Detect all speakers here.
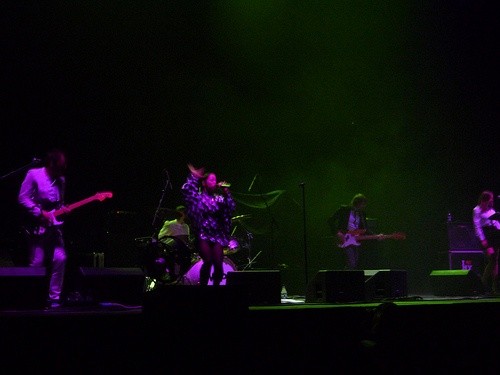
[78,266,145,307]
[226,270,282,307]
[305,269,408,305]
[429,221,488,297]
[0,266,49,311]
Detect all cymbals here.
[231,215,252,222]
[223,247,241,256]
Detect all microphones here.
[248,175,258,192]
[218,183,232,188]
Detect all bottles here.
[447,212,452,222]
[460,258,472,270]
[87,248,104,268]
[280,283,287,299]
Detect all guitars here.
[25,192,112,235]
[336,230,407,249]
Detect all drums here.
[183,258,239,285]
[158,235,192,258]
[152,258,180,281]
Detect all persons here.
[181,164,236,285]
[18,151,73,308]
[327,193,368,270]
[472,191,500,293]
[158,205,193,243]
